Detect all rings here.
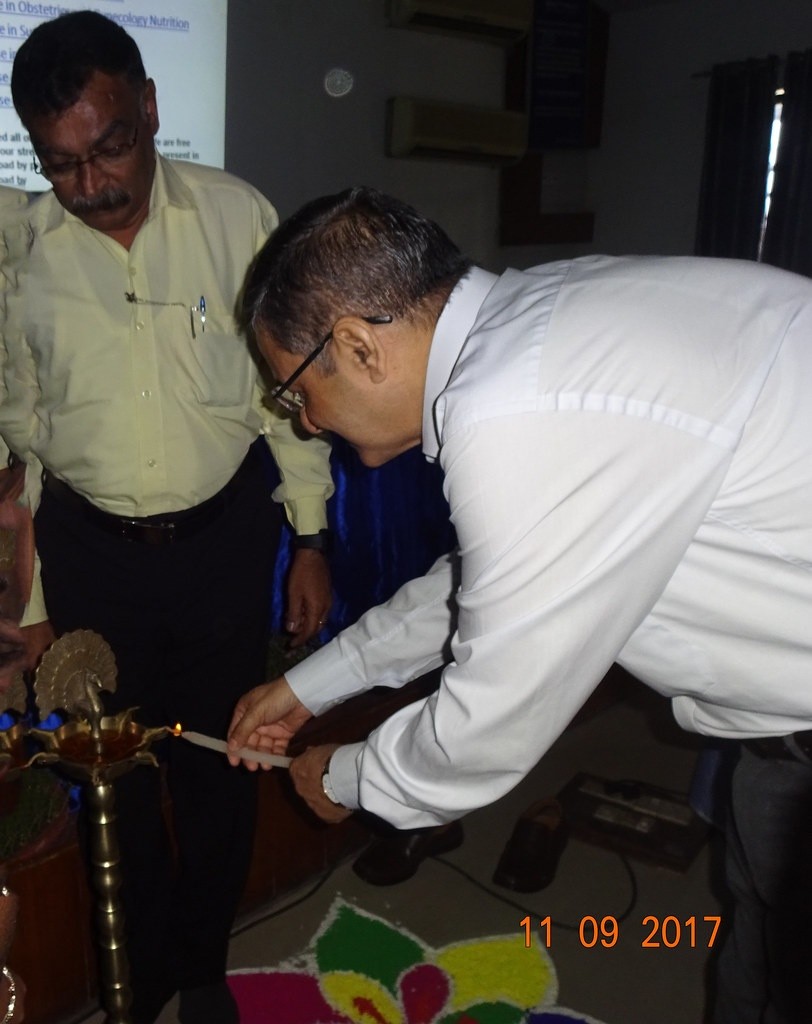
[318,621,326,624]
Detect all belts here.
[44,444,261,546]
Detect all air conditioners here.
[384,95,530,167]
[383,0,534,48]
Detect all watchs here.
[321,755,351,811]
[294,529,335,561]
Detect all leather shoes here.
[494,797,577,892]
[351,821,462,885]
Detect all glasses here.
[271,314,392,413]
[33,88,144,183]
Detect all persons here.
[227,188,812,1024]
[0,10,335,1023]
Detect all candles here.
[178,730,293,768]
